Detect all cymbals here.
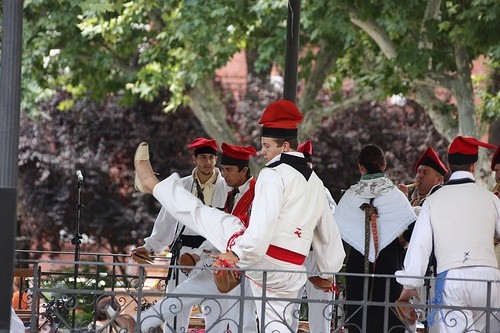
[214,267,241,293]
[395,301,418,326]
[131,248,153,265]
[180,253,195,273]
[308,276,332,291]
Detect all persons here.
[491,142,500,271]
[394,135,500,333]
[88,297,121,333]
[397,146,448,333]
[337,144,416,333]
[134,100,346,333]
[131,138,229,333]
[106,141,257,333]
[291,139,338,333]
[12,220,31,310]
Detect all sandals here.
[134,141,150,194]
[104,304,130,333]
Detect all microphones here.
[76,170,83,184]
[195,178,206,205]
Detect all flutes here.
[398,182,420,189]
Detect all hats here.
[221,142,257,168]
[415,146,448,177]
[258,100,303,139]
[491,144,500,171]
[447,136,497,166]
[187,138,218,158]
